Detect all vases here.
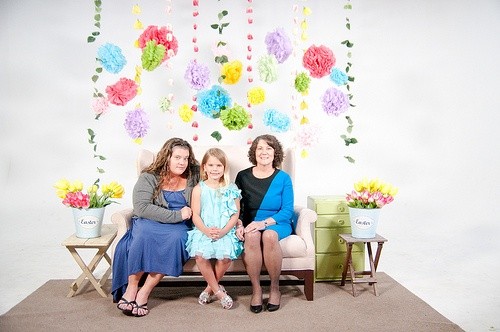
[72,207,105,239]
[349,207,380,239]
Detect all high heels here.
[249,288,263,314]
[267,290,282,312]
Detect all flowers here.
[54,178,124,210]
[346,181,398,209]
[87,0,359,173]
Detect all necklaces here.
[171,179,180,190]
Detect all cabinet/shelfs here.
[306,196,365,282]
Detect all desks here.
[62,227,118,298]
[338,233,388,298]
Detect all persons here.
[235,135,297,314]
[111,137,202,317]
[185,148,243,310]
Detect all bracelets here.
[264,220,267,227]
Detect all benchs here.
[111,206,317,301]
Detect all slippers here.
[116,297,149,318]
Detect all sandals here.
[214,284,233,310]
[197,288,212,306]
